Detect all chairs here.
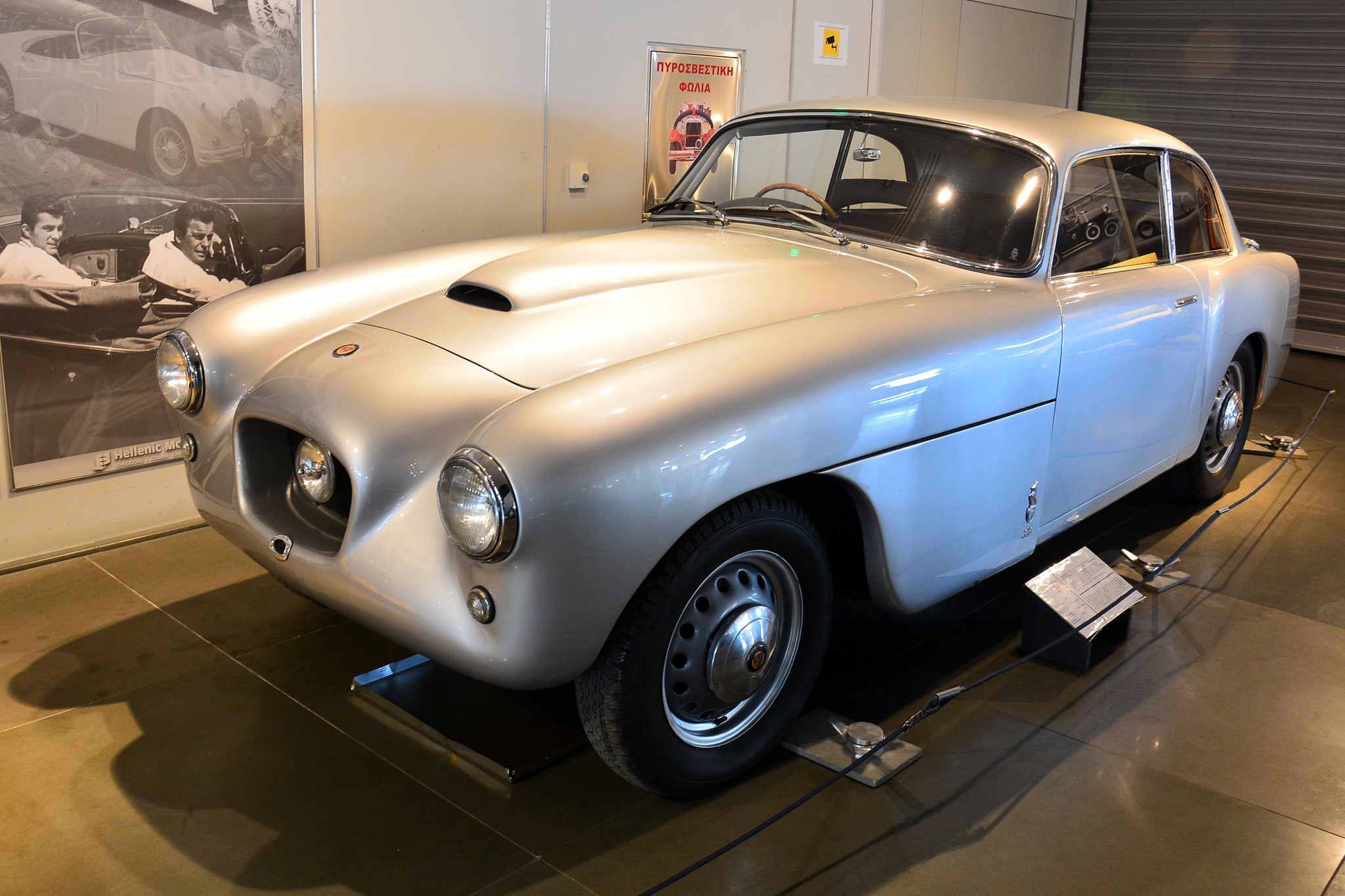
[913,191,1029,265]
[829,177,915,234]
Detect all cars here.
[0,1,304,187]
[151,90,1303,804]
[0,185,306,454]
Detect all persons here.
[0,194,117,288]
[140,200,251,305]
